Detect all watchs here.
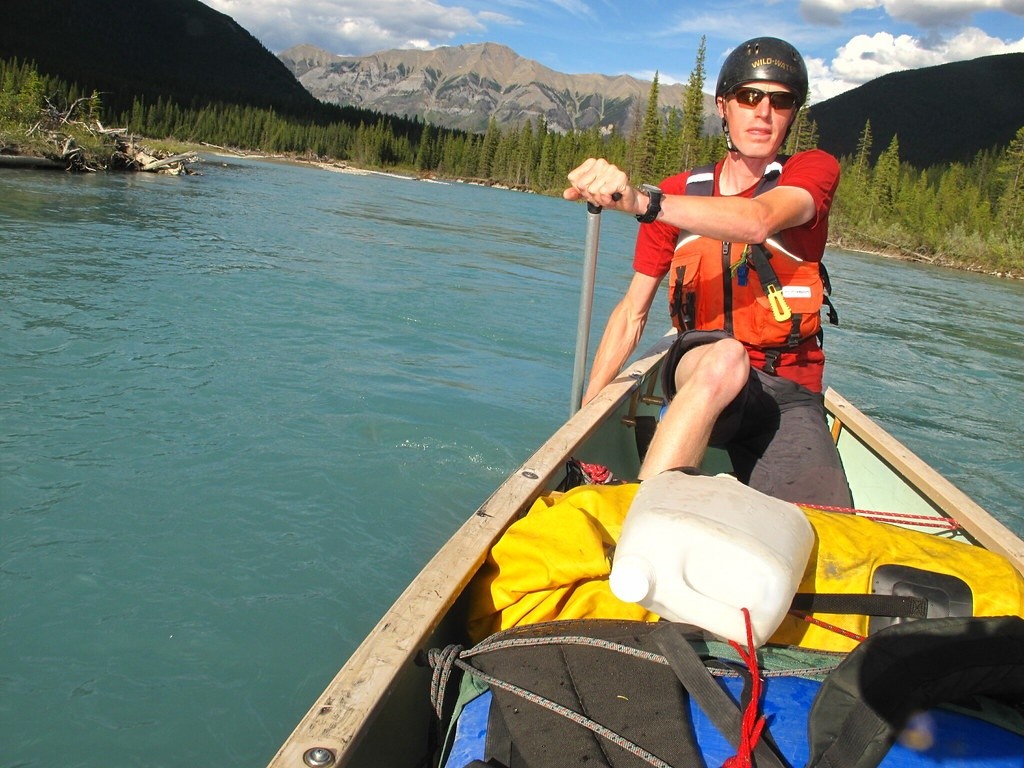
[635,184,663,223]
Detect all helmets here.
[716,37,809,108]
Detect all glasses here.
[731,86,797,108]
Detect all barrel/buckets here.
[609,471,815,650]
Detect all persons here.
[563,38,855,515]
